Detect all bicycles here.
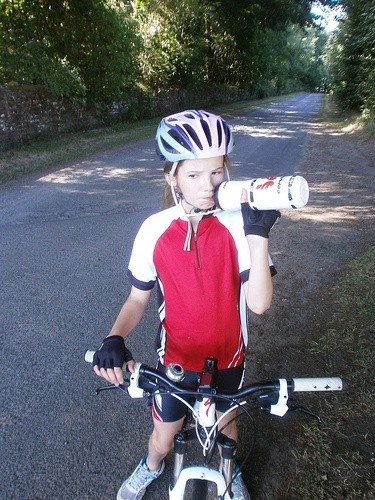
[85,349,345,500]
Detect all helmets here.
[155,110,235,161]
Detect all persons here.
[91,108,283,500]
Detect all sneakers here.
[116,455,164,500]
[219,459,251,499]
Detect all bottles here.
[214,174,312,212]
